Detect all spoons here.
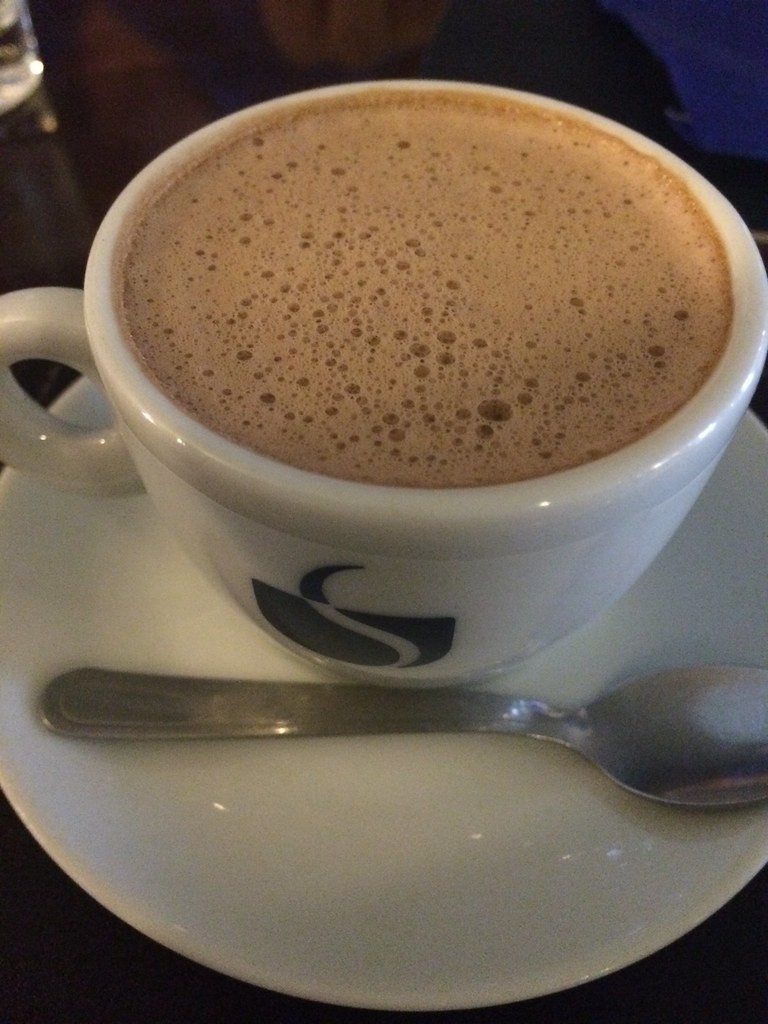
[37,664,768,809]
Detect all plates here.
[0,373,768,1011]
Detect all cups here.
[1,3,42,109]
[1,79,768,692]
